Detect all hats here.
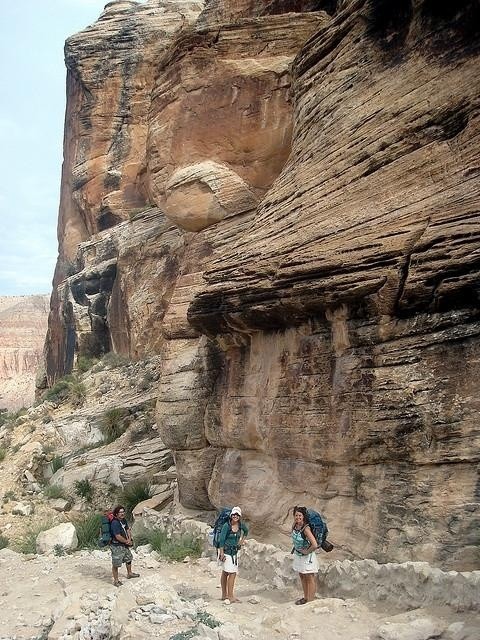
[230,506,242,518]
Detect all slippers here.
[222,597,241,603]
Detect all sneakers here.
[295,598,307,605]
[114,580,123,586]
[127,573,139,579]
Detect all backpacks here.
[213,508,241,551]
[292,508,328,549]
[97,514,120,547]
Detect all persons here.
[108,505,139,587]
[217,506,249,604]
[290,504,320,605]
